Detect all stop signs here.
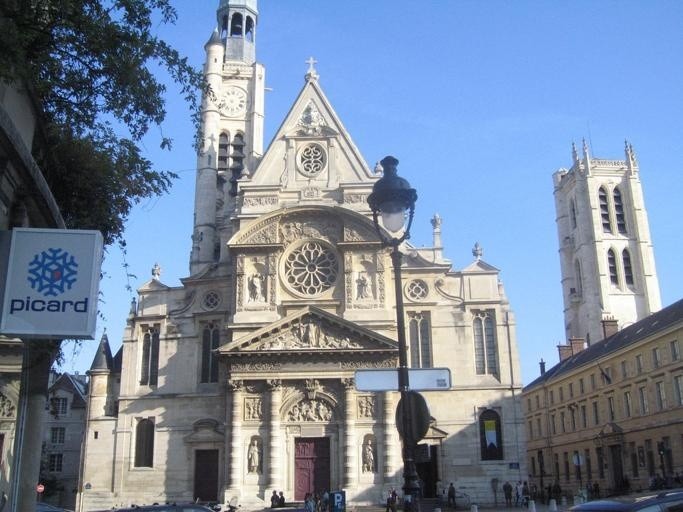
[37,485,44,493]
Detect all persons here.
[247,401,254,420]
[290,398,333,421]
[435,478,443,496]
[1,489,8,509]
[501,478,599,509]
[248,439,262,474]
[363,438,376,472]
[447,482,456,508]
[386,485,395,512]
[258,401,264,418]
[369,398,378,416]
[271,490,279,507]
[356,275,371,300]
[304,485,330,512]
[278,491,285,506]
[247,271,265,302]
[390,490,400,512]
[645,470,683,491]
[360,400,367,417]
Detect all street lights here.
[367,156,421,511]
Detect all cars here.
[569,490,683,511]
[110,504,220,512]
